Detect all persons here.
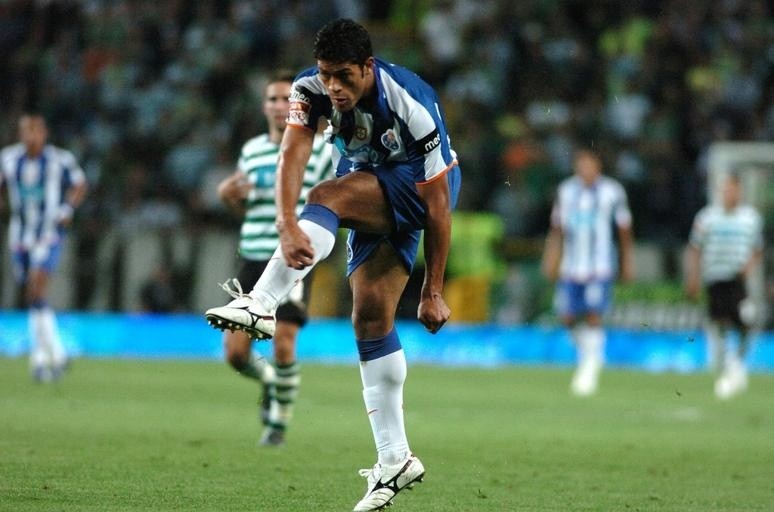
[201,19,461,511]
[679,167,765,397]
[218,73,337,449]
[538,140,636,398]
[0,0,773,333]
[1,112,87,383]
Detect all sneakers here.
[204,292,278,343]
[259,425,285,446]
[353,450,426,512]
[258,364,279,426]
